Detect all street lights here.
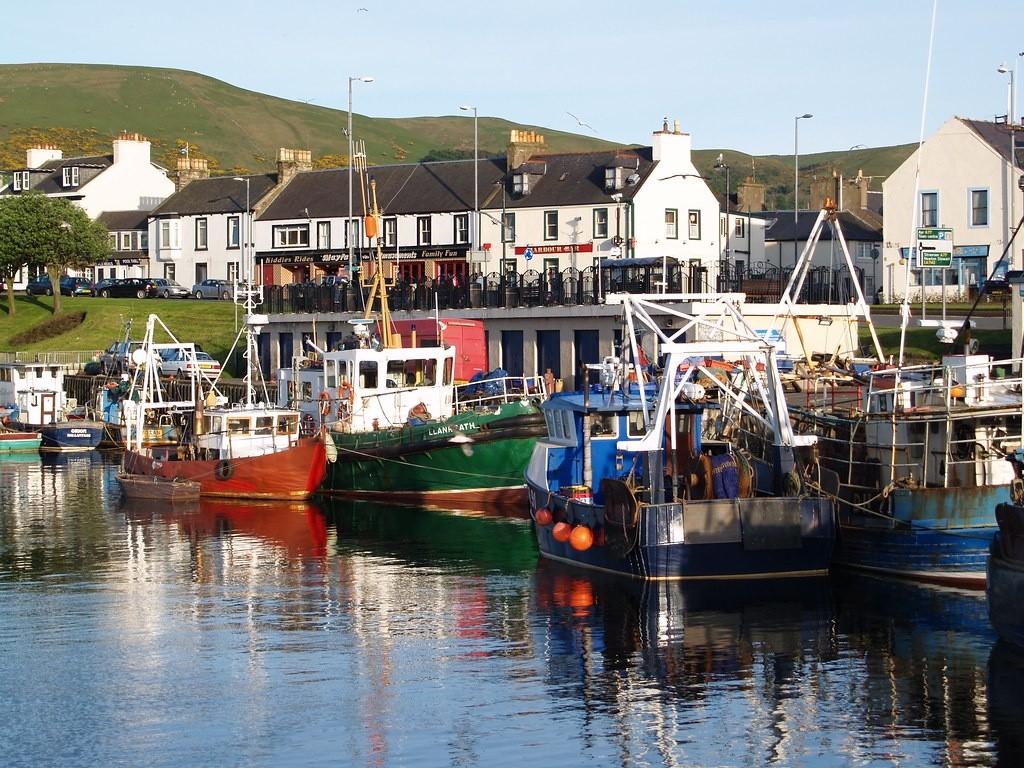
[996,62,1018,275]
[348,75,373,281]
[793,114,814,280]
[715,163,733,294]
[233,176,259,285]
[460,105,485,276]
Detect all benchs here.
[740,280,786,298]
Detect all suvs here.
[26,273,70,296]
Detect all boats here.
[1,415,42,452]
[121,310,332,506]
[525,279,845,607]
[772,203,1023,633]
[268,244,552,524]
[159,494,329,565]
[1,451,44,465]
[117,497,202,515]
[42,451,104,468]
[0,365,106,452]
[315,501,538,573]
[115,474,203,499]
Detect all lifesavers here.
[318,392,330,416]
[302,414,315,434]
[339,382,354,405]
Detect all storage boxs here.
[560,486,592,504]
[630,350,792,404]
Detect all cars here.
[152,279,191,299]
[92,278,123,298]
[52,277,94,297]
[193,278,245,301]
[103,279,158,298]
